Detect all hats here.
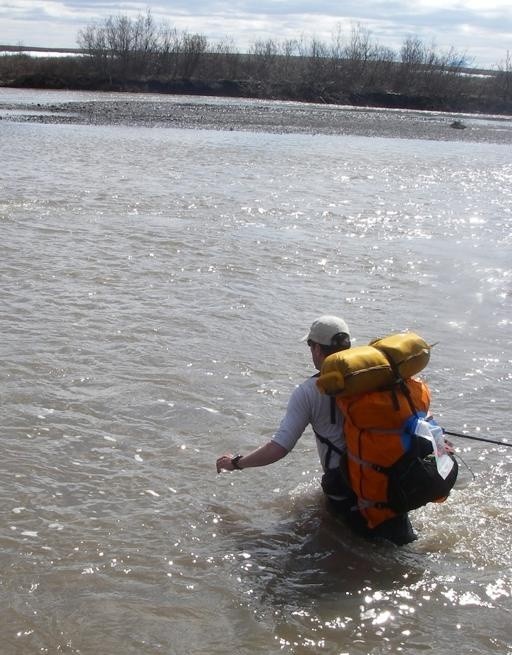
[298,312,351,349]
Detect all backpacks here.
[310,370,460,533]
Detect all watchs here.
[230,454,244,469]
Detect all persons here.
[216,314,455,545]
